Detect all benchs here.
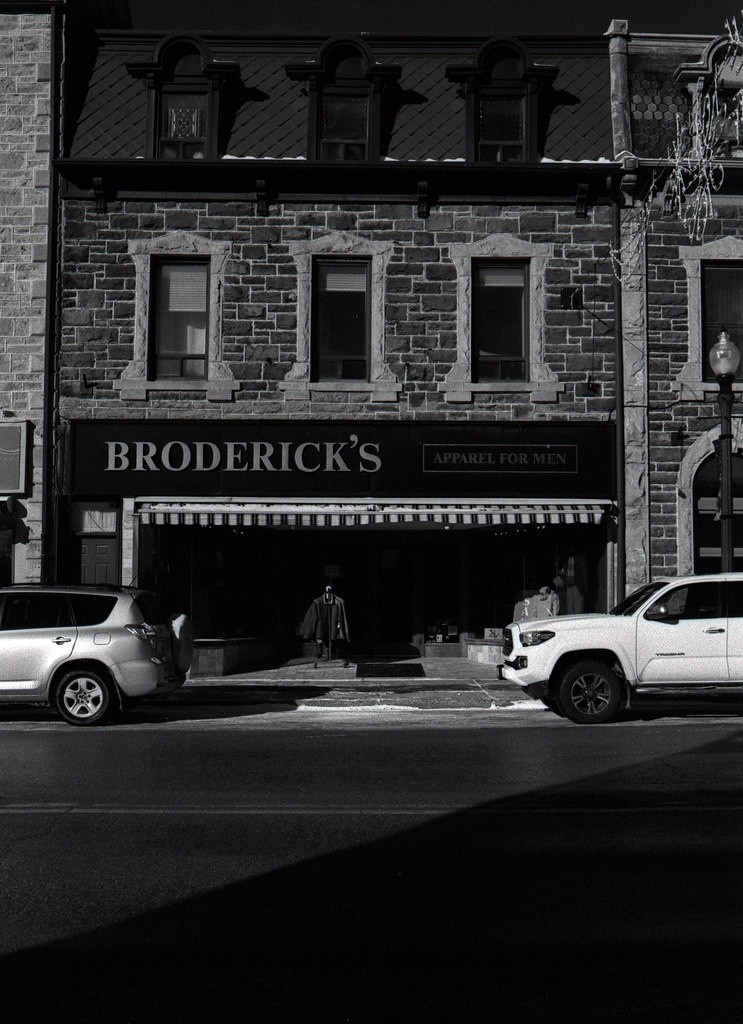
[190,637,256,678]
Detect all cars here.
[496,573,743,724]
[0,582,194,726]
